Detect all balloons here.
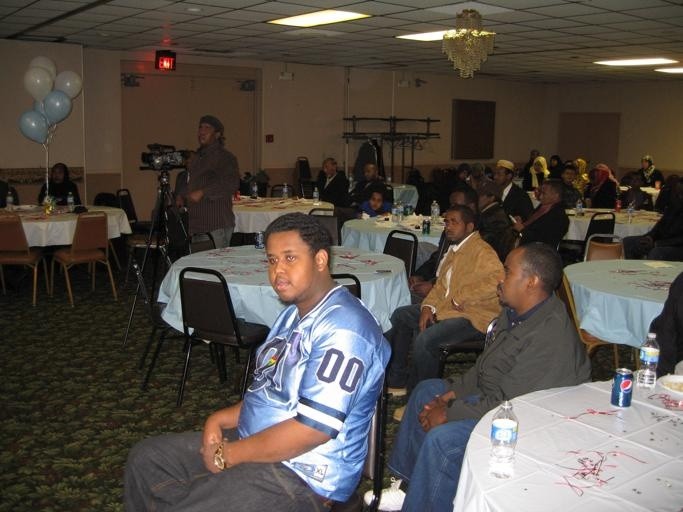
[16,54,83,145]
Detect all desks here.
[562,259,682,366]
[158,244,414,354]
[182,195,334,237]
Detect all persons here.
[382,207,506,421]
[448,149,618,247]
[386,184,501,388]
[360,240,592,512]
[619,173,654,210]
[172,149,197,238]
[622,178,682,261]
[642,271,683,384]
[635,155,664,186]
[0,180,9,208]
[36,161,84,205]
[316,157,393,219]
[172,115,239,252]
[120,210,393,511]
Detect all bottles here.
[6,191,13,212]
[281,181,287,200]
[627,203,634,223]
[636,332,660,394]
[575,198,582,216]
[391,198,440,225]
[313,186,319,204]
[66,192,75,212]
[250,181,260,198]
[489,396,518,479]
[615,193,622,212]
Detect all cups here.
[48,195,57,210]
[654,181,661,191]
[45,199,52,215]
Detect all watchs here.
[212,439,229,472]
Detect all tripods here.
[121,170,193,348]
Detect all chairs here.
[130,258,224,390]
[49,209,118,308]
[1,213,50,307]
[270,182,517,278]
[326,352,389,511]
[435,338,484,381]
[383,229,419,280]
[559,272,641,369]
[185,231,217,255]
[2,189,141,227]
[308,208,343,247]
[517,155,681,261]
[330,272,362,300]
[173,266,272,407]
[122,210,174,288]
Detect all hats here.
[498,160,514,170]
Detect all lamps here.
[441,7,495,81]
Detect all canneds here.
[404,204,413,216]
[422,219,430,234]
[255,231,265,249]
[611,368,633,407]
[234,190,241,201]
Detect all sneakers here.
[364,479,408,511]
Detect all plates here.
[657,373,682,396]
